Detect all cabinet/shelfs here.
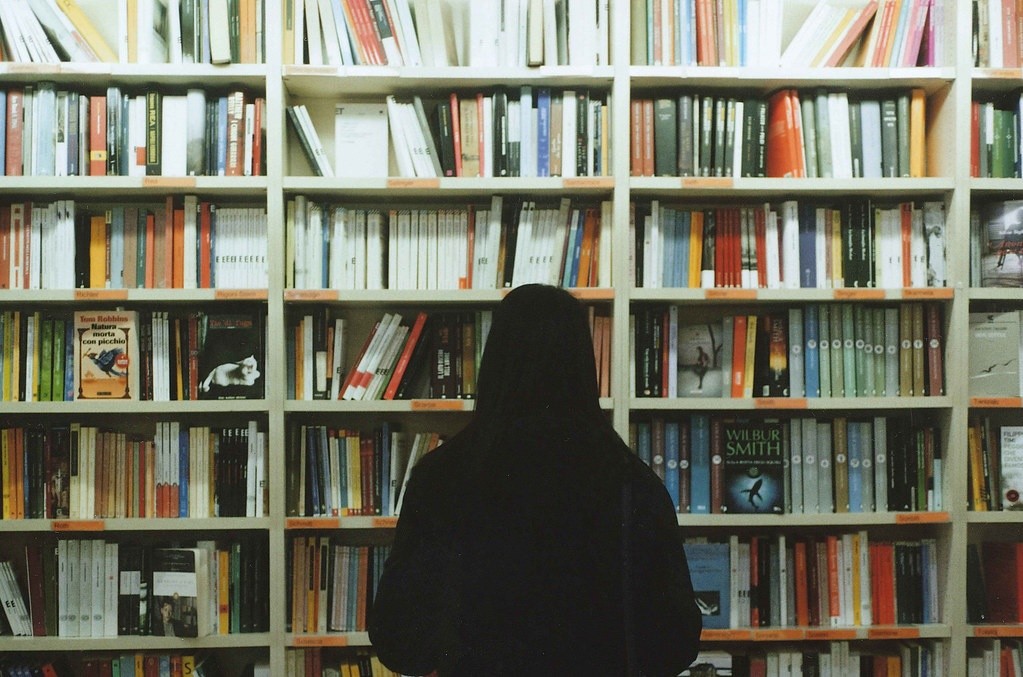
[1,0,1023,676]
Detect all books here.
[0,0,1023,677]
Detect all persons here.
[370,284,703,677]
